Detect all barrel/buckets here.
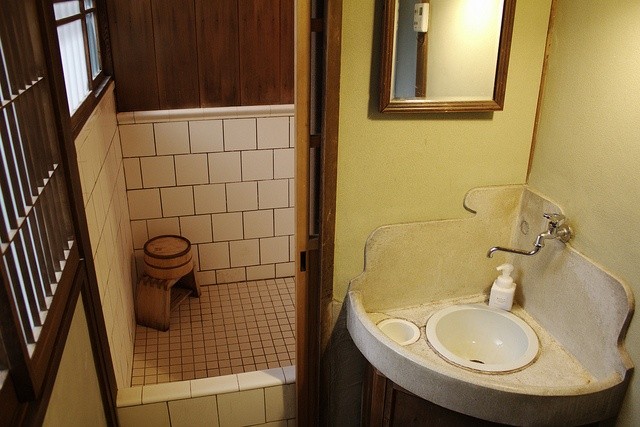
[143,234,193,279]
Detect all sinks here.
[426,304,539,373]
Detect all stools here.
[135,263,202,332]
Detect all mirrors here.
[377,0,516,115]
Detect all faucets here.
[487,213,573,258]
